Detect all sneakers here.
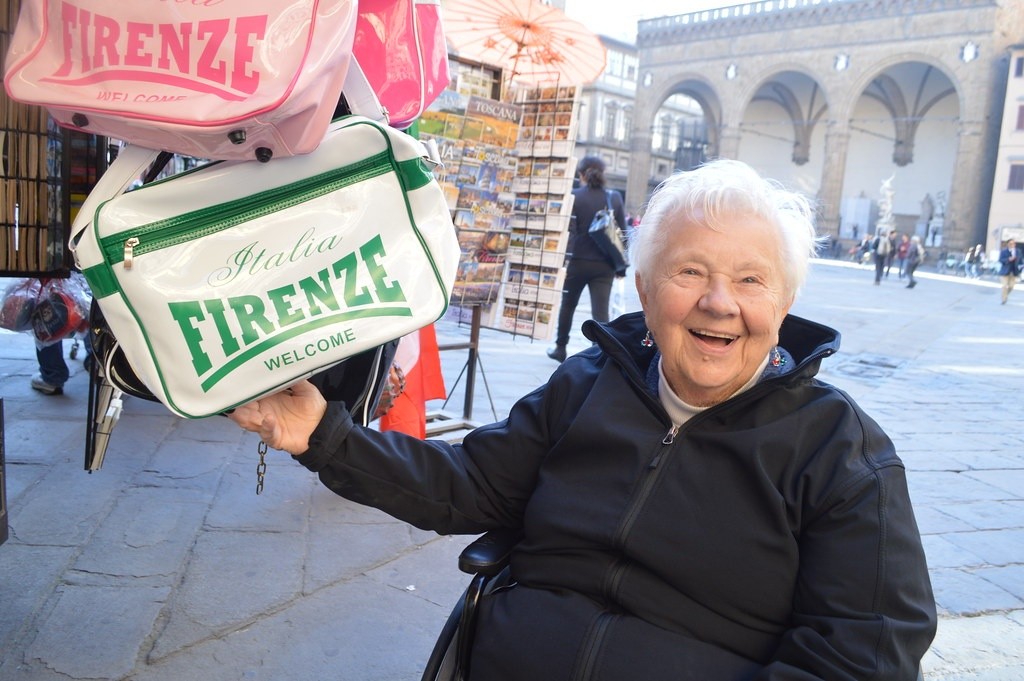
[31,371,63,394]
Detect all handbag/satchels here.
[588,187,630,274]
[65,53,460,420]
[0,271,92,343]
[3,1,359,162]
[93,320,406,430]
[353,0,451,129]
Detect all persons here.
[871,231,921,288]
[965,244,982,280]
[855,235,875,265]
[998,239,1022,304]
[548,156,632,362]
[626,212,641,226]
[233,158,939,681]
[31,278,93,395]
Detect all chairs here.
[421,527,526,681]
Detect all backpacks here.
[877,236,891,256]
[916,244,925,265]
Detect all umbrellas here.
[441,0,607,101]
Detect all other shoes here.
[875,273,882,282]
[1007,285,1013,294]
[906,281,916,288]
[1001,297,1008,304]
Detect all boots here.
[546,342,566,363]
